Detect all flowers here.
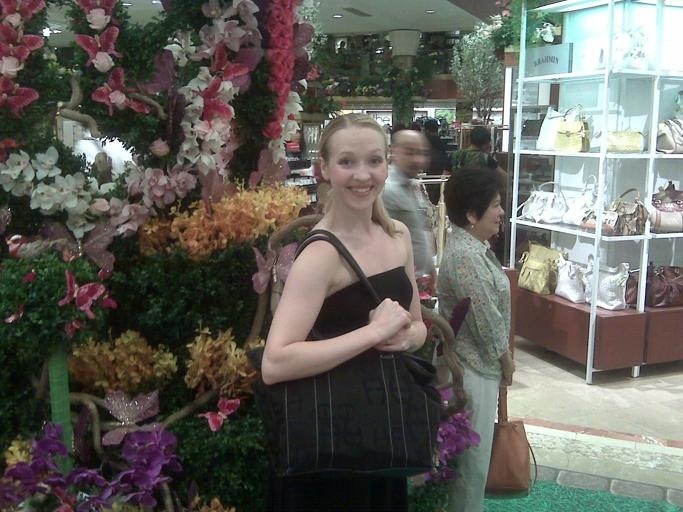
[0,0,320,470]
[489,0,512,48]
[68,185,316,511]
[431,410,481,484]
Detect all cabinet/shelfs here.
[510,0,683,384]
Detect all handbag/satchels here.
[535,103,583,151]
[625,264,682,309]
[252,231,441,484]
[560,174,607,226]
[605,129,644,153]
[515,178,569,224]
[554,248,590,304]
[650,180,683,234]
[517,251,549,295]
[656,118,683,154]
[584,258,630,311]
[528,240,560,290]
[579,206,620,235]
[603,187,648,237]
[482,380,536,496]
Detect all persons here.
[381,129,436,303]
[436,166,514,512]
[443,127,509,177]
[262,114,439,512]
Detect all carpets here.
[482,478,683,511]
